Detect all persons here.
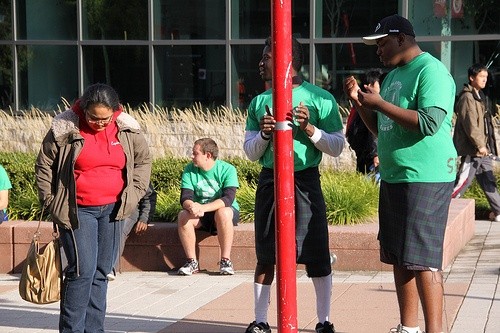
[0,163,13,224]
[35,84,156,333]
[346,17,456,333]
[243,36,346,333]
[348,64,500,224]
[177,138,240,275]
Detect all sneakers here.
[177,258,199,276]
[217,258,234,275]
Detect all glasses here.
[86,110,114,123]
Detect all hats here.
[362,14,416,46]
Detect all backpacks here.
[345,103,372,155]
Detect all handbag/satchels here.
[19,194,63,304]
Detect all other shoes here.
[315,321,335,333]
[245,321,271,333]
[388,324,425,333]
